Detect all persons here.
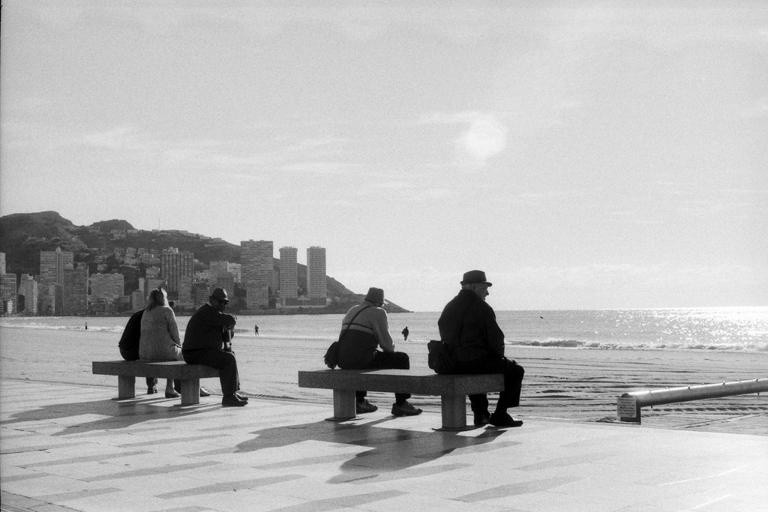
[438,270,525,428]
[182,287,248,407]
[402,326,409,341]
[335,287,423,417]
[254,324,259,336]
[138,288,210,398]
[118,300,181,395]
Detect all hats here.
[363,287,389,305]
[459,269,492,288]
[208,286,230,303]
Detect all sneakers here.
[199,387,211,397]
[390,402,422,415]
[147,384,158,395]
[356,400,378,414]
[222,391,249,406]
[165,387,181,399]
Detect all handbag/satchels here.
[323,341,340,369]
[427,338,459,375]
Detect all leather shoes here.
[473,411,490,427]
[489,412,523,427]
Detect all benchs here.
[297,367,505,432]
[91,359,220,406]
